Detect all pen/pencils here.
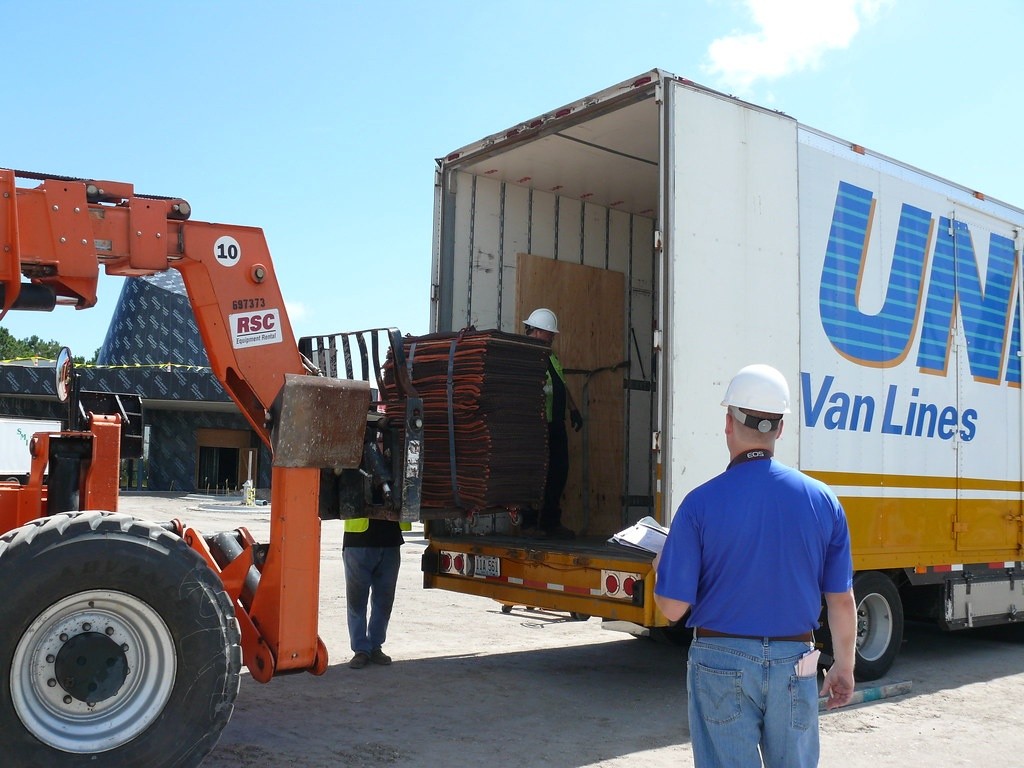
[822,667,835,699]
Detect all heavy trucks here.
[419,67,1023,684]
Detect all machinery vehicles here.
[1,163,539,768]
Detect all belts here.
[694,625,815,641]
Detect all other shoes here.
[369,650,392,665]
[349,652,370,669]
[542,521,577,539]
[515,522,552,540]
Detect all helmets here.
[522,308,560,334]
[720,363,792,417]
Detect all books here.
[604,515,671,558]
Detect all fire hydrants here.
[241,479,253,506]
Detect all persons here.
[649,363,859,768]
[343,517,411,669]
[521,307,585,541]
